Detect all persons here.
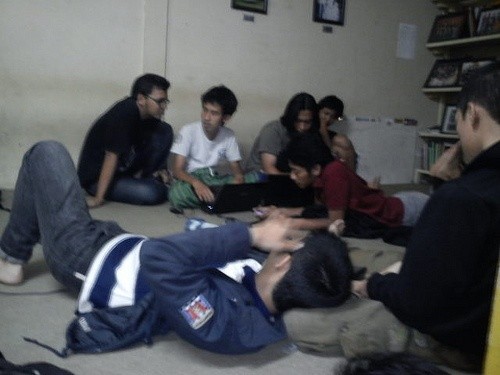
[77,73,174,207]
[244,63,500,375]
[169,84,243,211]
[0,140,354,357]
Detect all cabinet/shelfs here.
[416,0,500,190]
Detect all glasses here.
[144,94,170,106]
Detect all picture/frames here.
[441,103,458,134]
[474,7,500,35]
[424,60,462,87]
[313,0,346,26]
[428,11,467,41]
[230,0,268,14]
[458,58,497,88]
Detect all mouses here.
[170,207,184,214]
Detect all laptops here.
[200,182,270,214]
